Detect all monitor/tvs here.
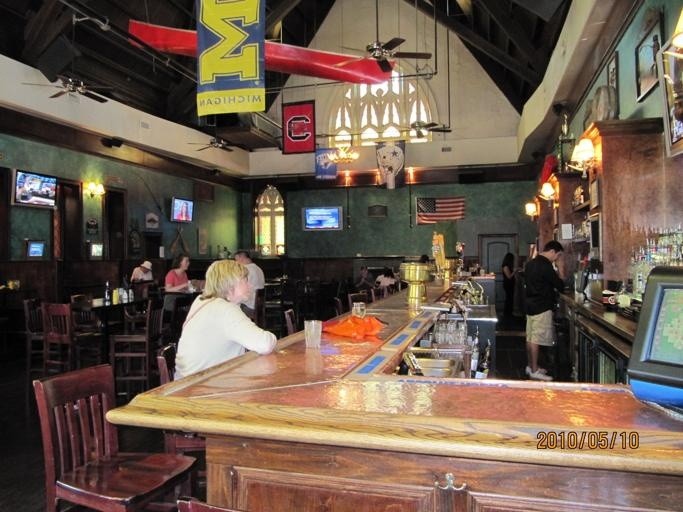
[301,206,343,231]
[626,266,682,408]
[12,168,59,209]
[171,196,195,223]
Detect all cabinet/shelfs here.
[537,117,683,383]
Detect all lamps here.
[325,143,361,164]
[84,181,106,199]
[538,182,555,208]
[525,202,537,222]
[670,8,683,50]
[569,138,596,179]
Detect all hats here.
[140,261,152,271]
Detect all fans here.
[22,16,116,106]
[186,126,247,152]
[330,0,433,74]
[410,121,452,139]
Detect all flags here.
[374,139,407,190]
[282,100,318,155]
[313,149,338,180]
[414,196,466,227]
[195,0,267,117]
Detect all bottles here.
[627,224,683,302]
[104,280,111,308]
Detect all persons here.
[163,253,199,312]
[167,257,281,381]
[501,251,523,320]
[374,266,397,292]
[352,263,376,293]
[130,260,154,284]
[233,250,267,315]
[522,240,569,381]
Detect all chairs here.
[32,364,198,512]
[70,294,97,330]
[23,298,62,369]
[156,343,207,501]
[109,298,165,372]
[170,294,196,333]
[348,293,369,312]
[176,496,240,512]
[334,297,343,316]
[284,308,297,336]
[371,288,385,302]
[262,283,294,329]
[72,305,108,369]
[40,302,72,375]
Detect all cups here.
[304,320,322,347]
[351,302,365,318]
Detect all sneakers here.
[529,371,554,382]
[525,367,548,374]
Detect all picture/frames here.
[655,37,683,160]
[633,14,665,104]
[606,50,620,115]
